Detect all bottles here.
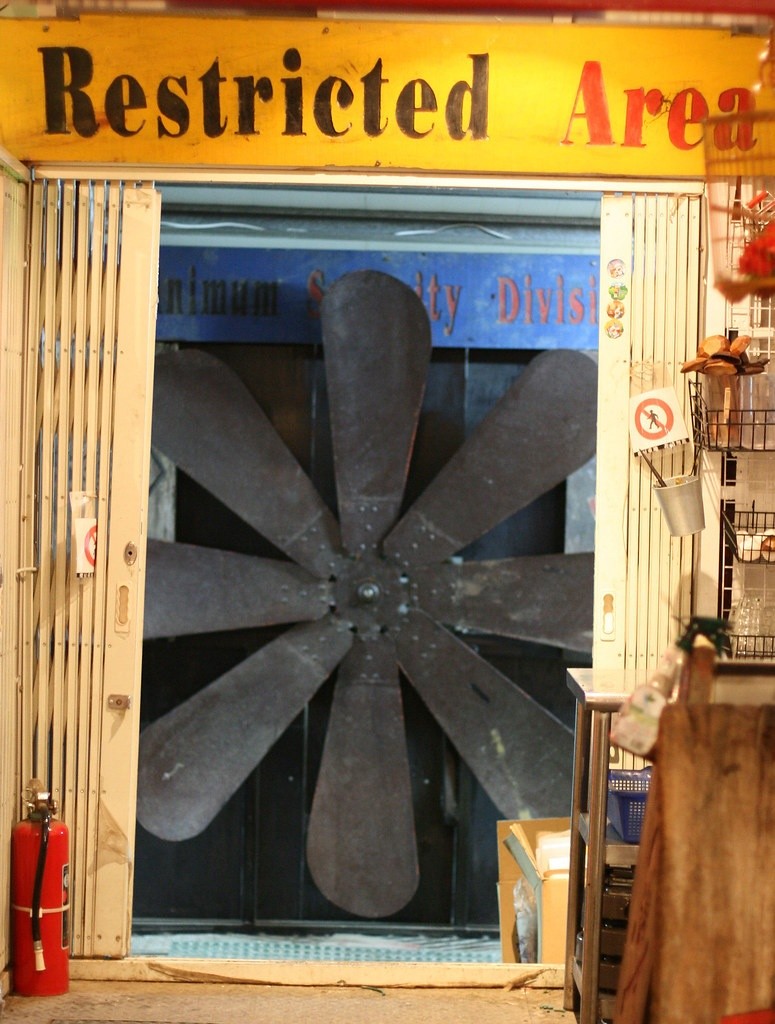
[608,674,673,758]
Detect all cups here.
[739,373,766,443]
[705,374,737,446]
[731,591,768,649]
[653,476,704,536]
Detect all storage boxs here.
[497,817,572,965]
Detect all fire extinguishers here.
[11,778,72,998]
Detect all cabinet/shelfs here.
[563,668,654,1024]
[687,378,775,677]
